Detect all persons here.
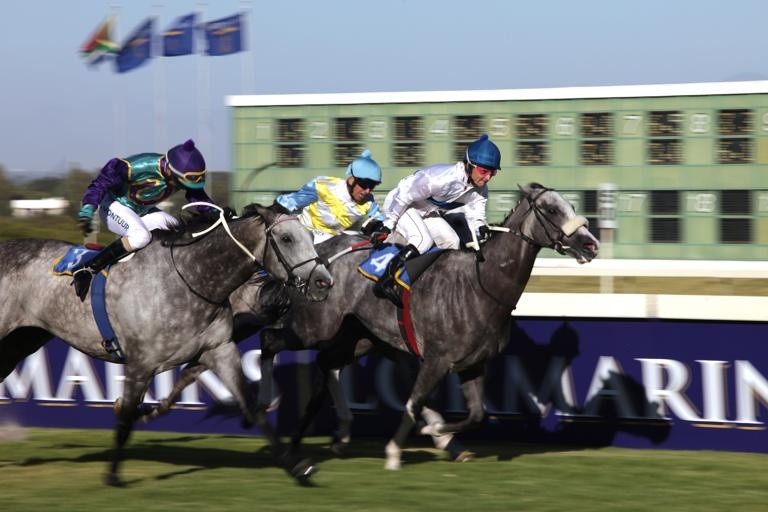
[272,151,387,245]
[372,135,501,309]
[71,141,235,296]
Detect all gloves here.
[369,225,391,250]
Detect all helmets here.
[345,149,382,185]
[461,133,502,171]
[164,139,205,191]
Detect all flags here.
[81,15,118,69]
[203,12,243,56]
[162,14,194,55]
[113,16,151,73]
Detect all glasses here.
[354,177,378,190]
[171,168,208,184]
[469,164,497,177]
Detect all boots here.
[71,235,130,298]
[372,245,419,308]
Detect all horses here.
[114,270,477,463]
[242,182,600,473]
[0,200,334,487]
